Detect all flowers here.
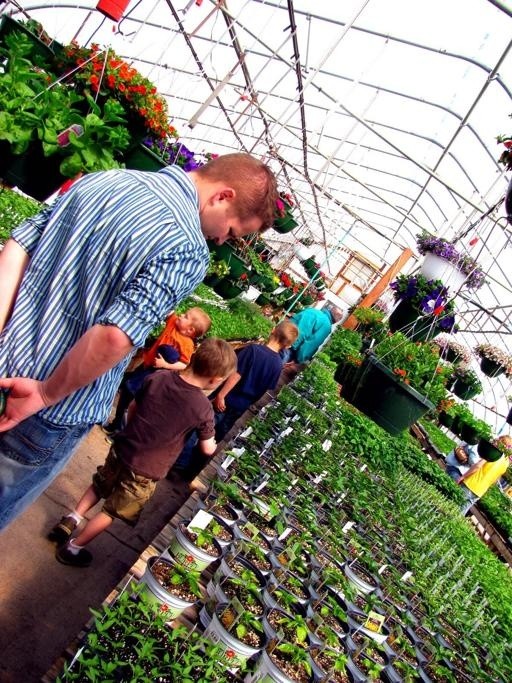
[0,14,512,683]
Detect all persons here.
[45,334,239,570]
[1,151,284,536]
[276,306,344,367]
[445,437,483,480]
[101,305,213,448]
[167,319,299,472]
[459,435,511,518]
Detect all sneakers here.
[55,548,94,567]
[53,514,78,538]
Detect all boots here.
[104,433,118,445]
[102,405,128,433]
